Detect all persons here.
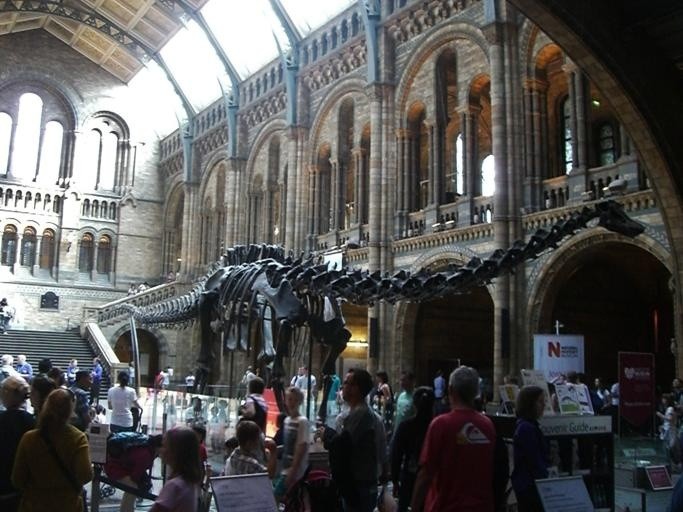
[126,270,179,296]
[0,352,683,511]
[0,296,16,335]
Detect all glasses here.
[60,385,75,400]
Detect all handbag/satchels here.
[246,394,266,427]
